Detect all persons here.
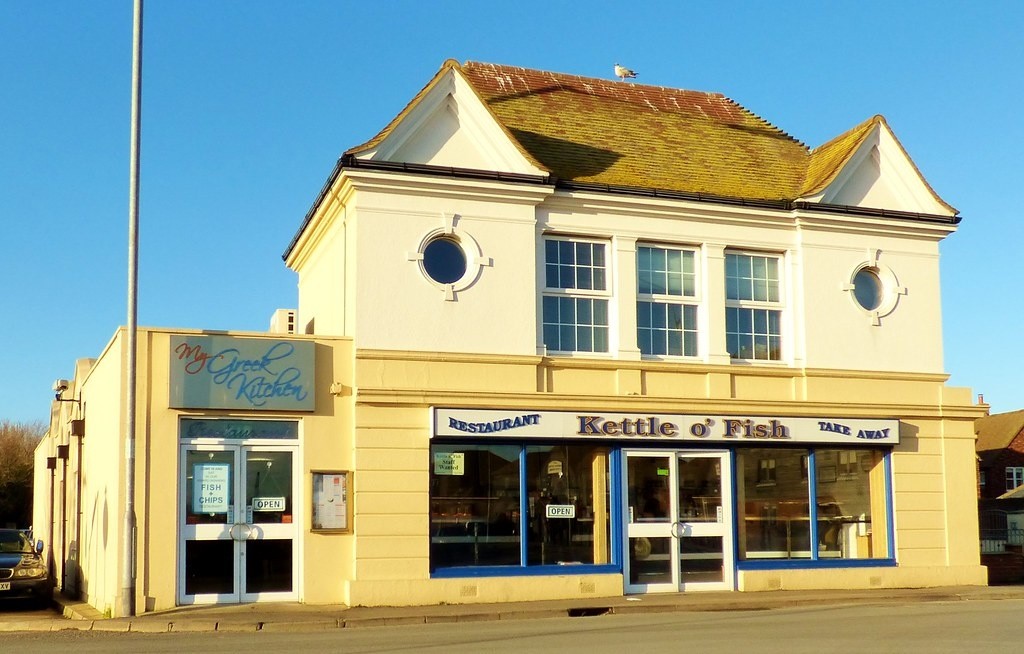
[644,495,666,517]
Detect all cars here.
[0,529,54,608]
